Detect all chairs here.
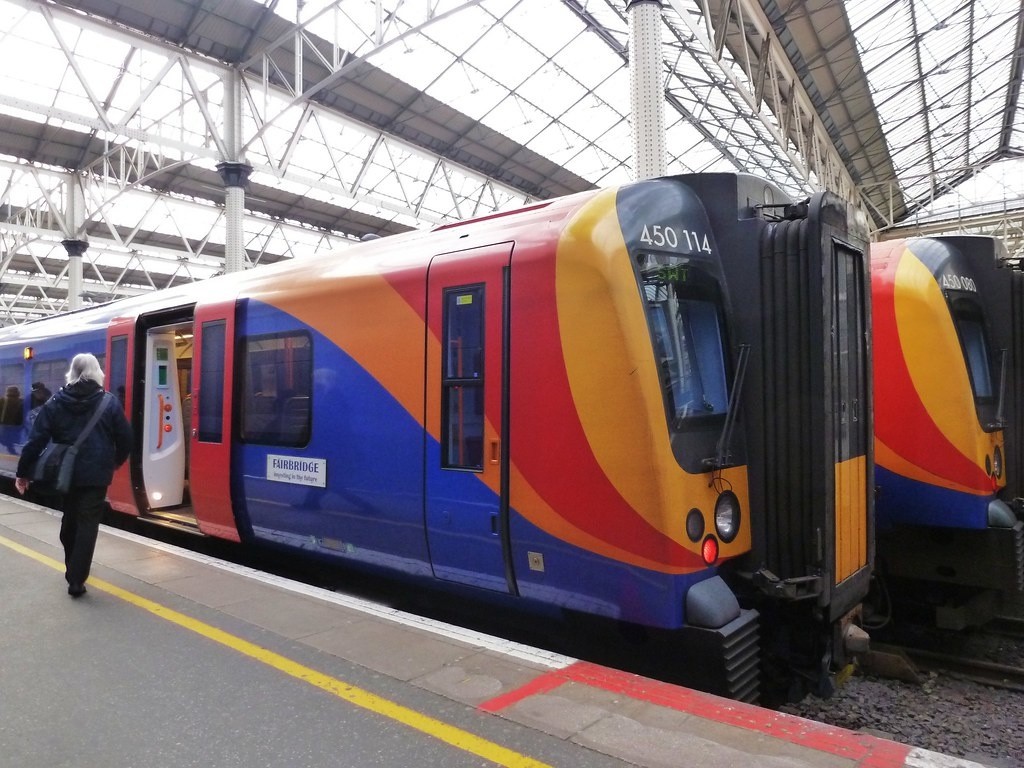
[182,393,310,465]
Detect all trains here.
[862,235,1024,641]
[0,173,878,709]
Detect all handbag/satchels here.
[33,440,79,494]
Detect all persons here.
[0,386,24,426]
[15,353,131,597]
[31,382,52,410]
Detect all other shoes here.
[68,582,86,597]
